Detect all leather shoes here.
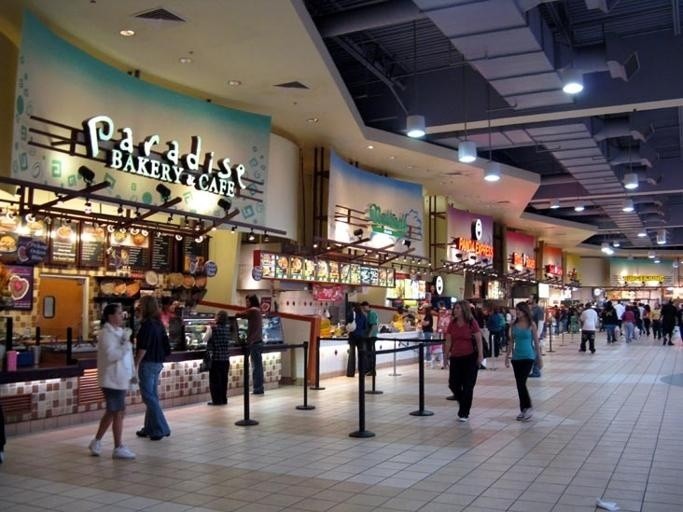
[136,426,171,441]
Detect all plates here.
[144,270,158,285]
[167,272,207,287]
[99,279,139,296]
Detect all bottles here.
[30,346,40,363]
[335,323,340,336]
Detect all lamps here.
[404,19,426,139]
[307,225,582,295]
[547,44,681,271]
[482,81,501,183]
[456,54,477,164]
[0,165,271,245]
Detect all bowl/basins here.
[56,227,72,241]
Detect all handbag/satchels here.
[199,350,213,373]
[471,334,490,356]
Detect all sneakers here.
[577,348,596,354]
[89,437,102,456]
[455,413,468,422]
[526,374,542,378]
[208,399,227,405]
[249,388,263,394]
[112,446,135,459]
[515,407,536,421]
[662,338,675,346]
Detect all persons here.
[159,296,176,334]
[345,303,371,377]
[419,298,683,422]
[359,302,378,376]
[201,311,229,405]
[88,304,137,459]
[135,295,171,440]
[235,295,264,394]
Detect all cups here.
[6,351,16,371]
